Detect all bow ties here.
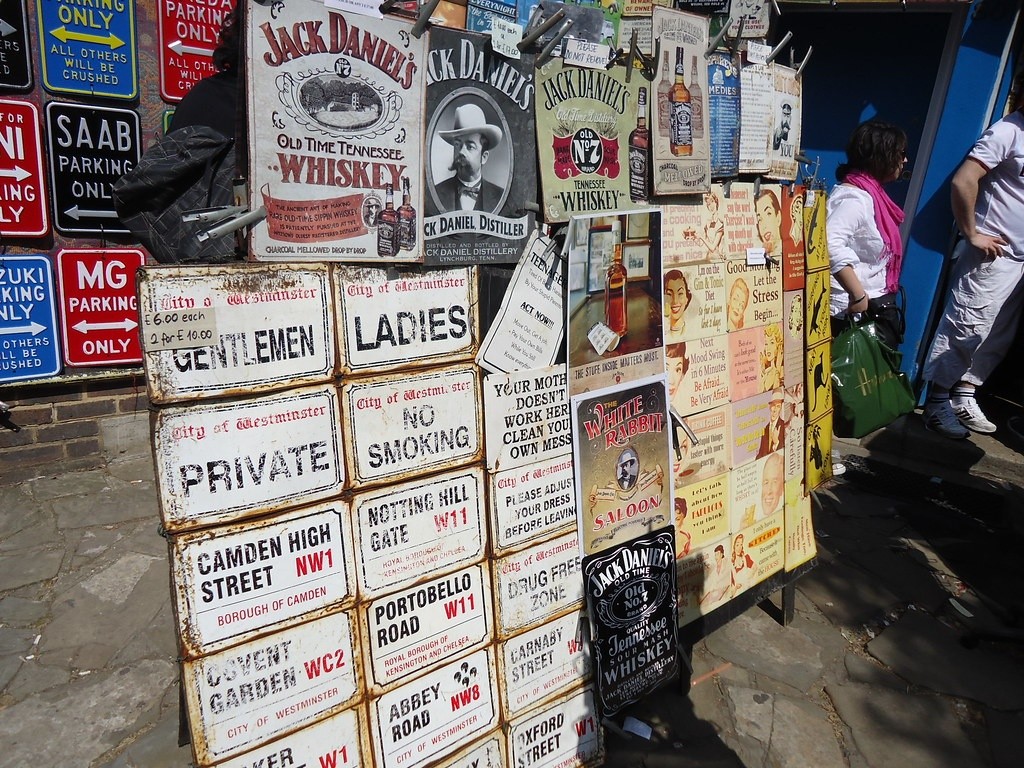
[456,185,479,194]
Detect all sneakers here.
[950,398,997,432]
[921,401,971,439]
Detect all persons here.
[429,103,508,219]
[816,119,906,475]
[920,93,1024,440]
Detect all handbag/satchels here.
[829,309,916,439]
[111,125,237,263]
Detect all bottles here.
[603,221,629,338]
[687,56,703,139]
[659,51,669,138]
[669,45,692,157]
[396,178,416,252]
[377,183,400,258]
[630,88,649,203]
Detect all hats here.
[438,104,502,151]
[618,453,636,466]
[365,199,380,206]
[768,387,784,403]
